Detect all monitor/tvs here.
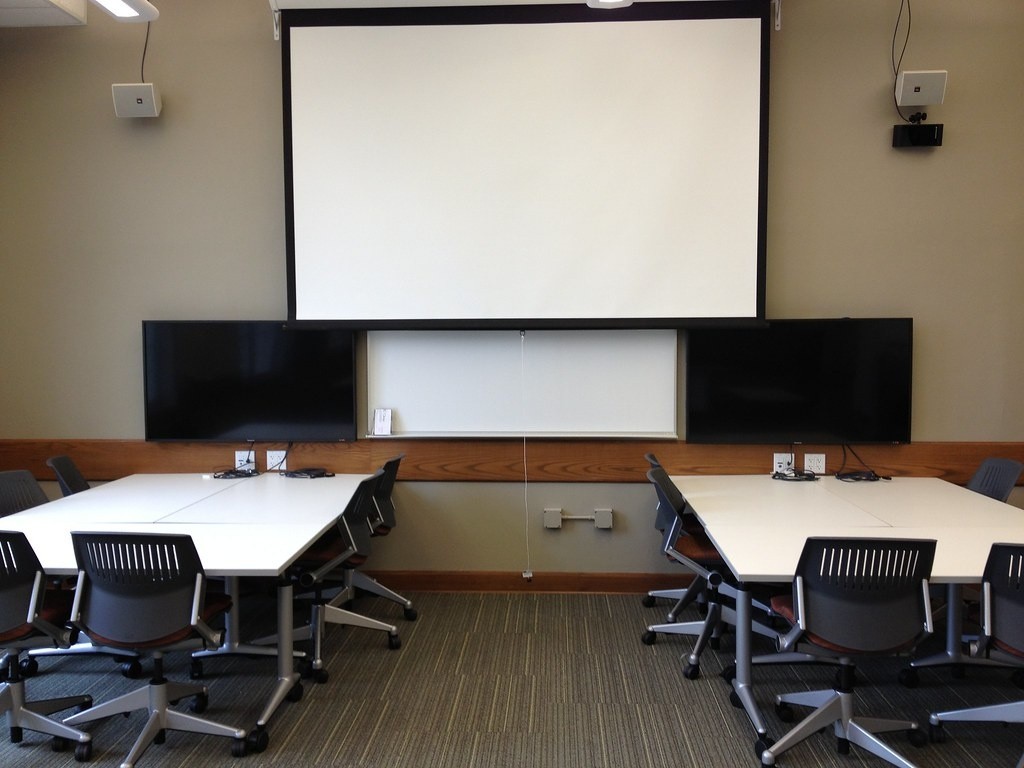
[684,318,913,445]
[140,320,357,443]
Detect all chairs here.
[640,452,1024,768]
[0,455,418,768]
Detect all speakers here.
[894,70,947,106]
[111,82,162,118]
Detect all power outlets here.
[266,450,288,471]
[234,450,255,470]
[773,453,796,474]
[804,454,826,474]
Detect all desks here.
[0,469,376,752]
[670,474,1024,761]
